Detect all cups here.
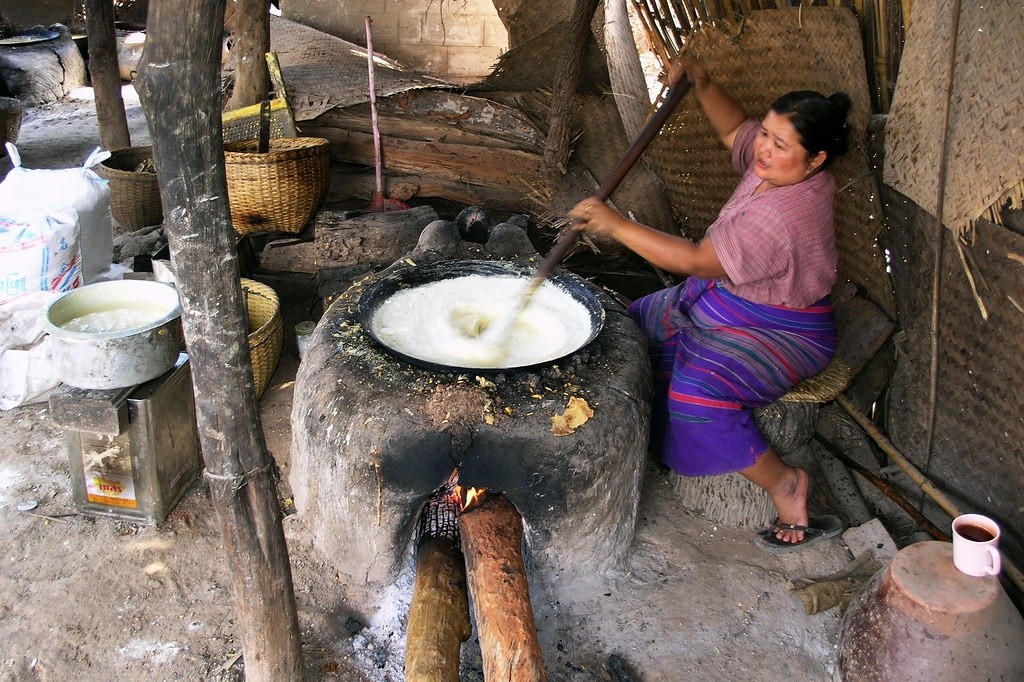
[951,513,1002,577]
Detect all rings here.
[585,205,594,213]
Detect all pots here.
[356,257,606,372]
[38,279,183,390]
[0,29,61,47]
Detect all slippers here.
[754,515,843,554]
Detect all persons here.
[568,55,852,553]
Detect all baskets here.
[96,145,164,232]
[221,138,330,234]
[0,97,24,158]
[177,276,283,401]
